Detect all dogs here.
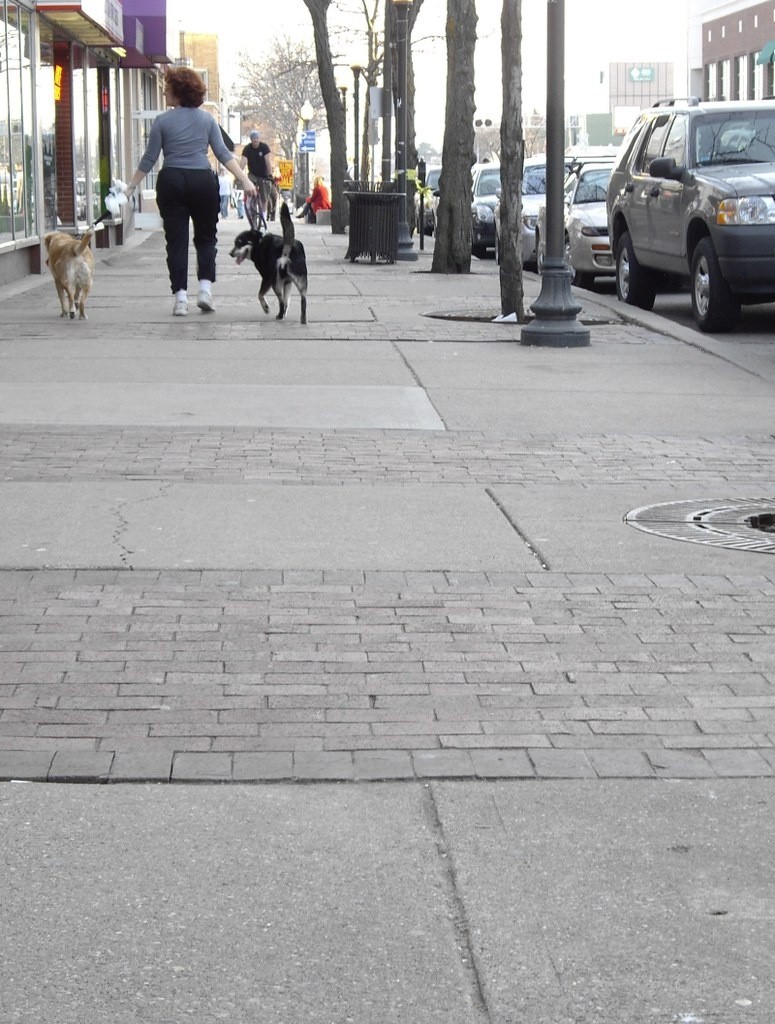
[228,202,308,324]
[45,227,94,320]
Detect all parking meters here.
[417,157,427,250]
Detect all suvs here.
[605,97,775,331]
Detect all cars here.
[415,163,440,236]
[75,178,102,220]
[536,160,619,289]
[494,158,551,269]
[472,164,501,258]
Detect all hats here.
[250,130,260,138]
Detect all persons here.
[295,176,332,224]
[24,134,33,236]
[119,66,258,317]
[483,158,490,164]
[216,131,283,221]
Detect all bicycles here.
[243,180,268,230]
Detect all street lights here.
[346,44,367,181]
[300,99,315,200]
[335,67,350,112]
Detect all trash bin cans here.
[344,180,395,260]
[342,192,406,264]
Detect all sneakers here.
[196,290,216,311]
[172,300,190,315]
